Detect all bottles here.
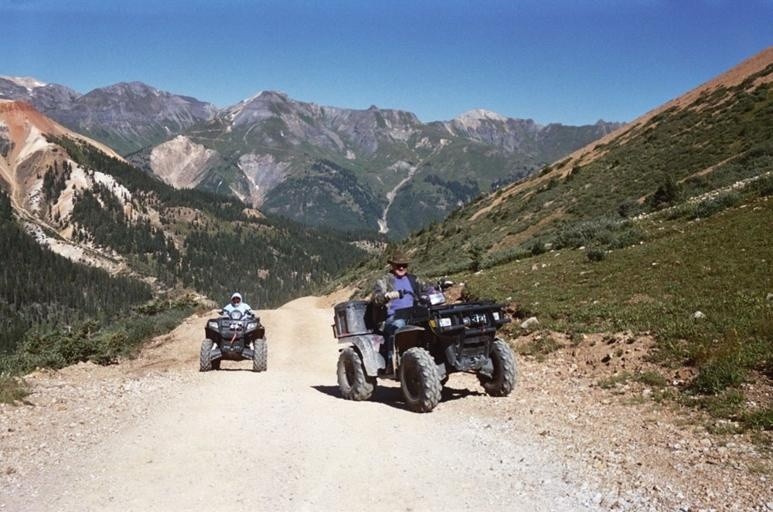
[387,251,413,264]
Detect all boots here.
[330,278,519,413]
[199,307,268,373]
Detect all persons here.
[371,255,453,334]
[216,292,256,320]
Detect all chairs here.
[385,351,394,375]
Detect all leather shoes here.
[443,281,453,291]
[385,291,399,300]
